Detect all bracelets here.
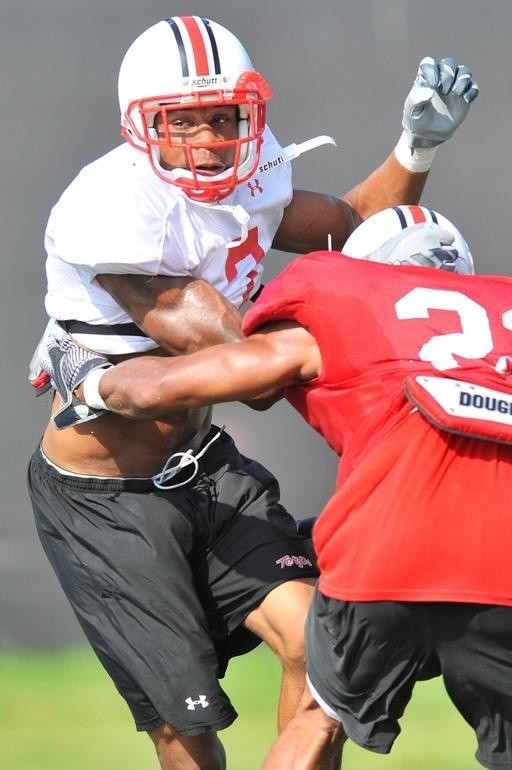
[83,369,112,412]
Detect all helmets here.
[117,16,273,204]
[341,204,475,277]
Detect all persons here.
[28,15,477,770]
[29,206,512,769]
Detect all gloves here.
[402,57,479,148]
[29,332,113,431]
[367,222,458,272]
